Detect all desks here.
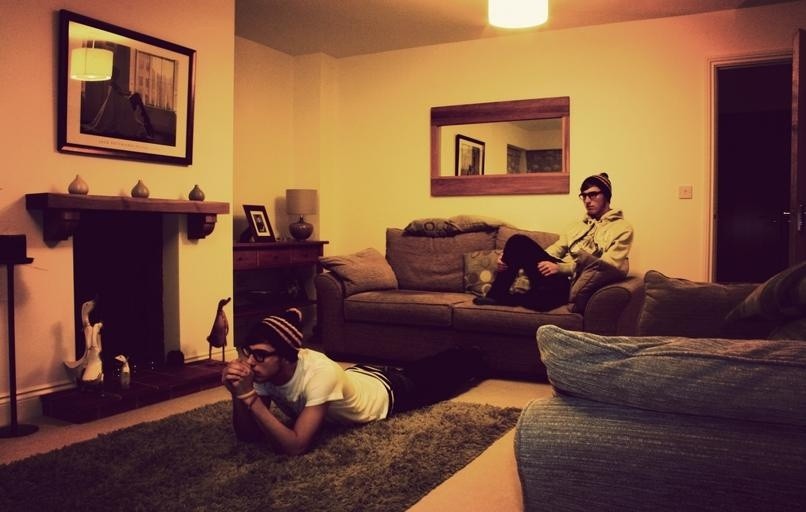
[233,238,329,347]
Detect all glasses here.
[579,191,603,200]
[241,344,276,363]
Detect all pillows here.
[636,270,749,339]
[450,215,504,232]
[385,228,496,291]
[405,218,463,237]
[568,260,627,313]
[464,250,503,295]
[537,324,806,426]
[496,225,560,251]
[726,262,806,342]
[317,247,398,296]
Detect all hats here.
[255,307,303,362]
[580,173,611,197]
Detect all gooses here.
[207,297,232,362]
[64,301,104,387]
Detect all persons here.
[472,172,633,313]
[222,302,487,456]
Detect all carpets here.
[0,396,523,512]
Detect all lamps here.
[70,40,114,82]
[285,188,318,240]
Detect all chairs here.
[515,263,806,512]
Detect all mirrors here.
[430,96,571,197]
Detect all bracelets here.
[233,388,260,410]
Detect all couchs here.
[318,272,644,386]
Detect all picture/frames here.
[242,204,276,242]
[455,134,485,176]
[56,8,196,165]
